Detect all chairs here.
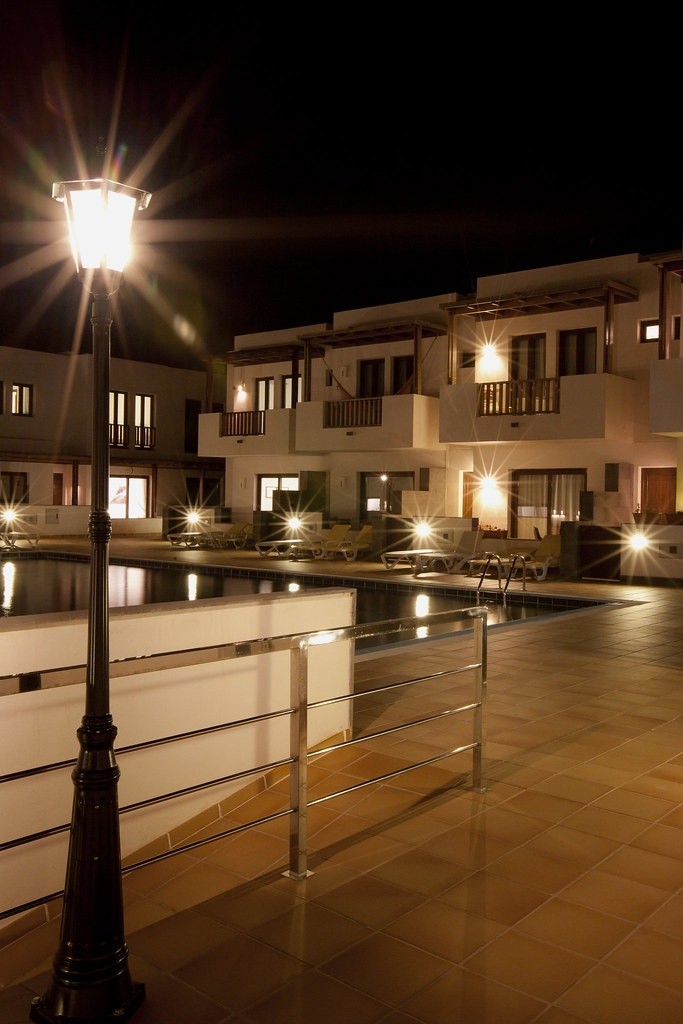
[500,535,561,580]
[0,522,39,546]
[168,521,374,563]
[419,532,483,572]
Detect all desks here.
[256,539,304,555]
[181,531,223,547]
[467,559,514,575]
[381,549,432,570]
